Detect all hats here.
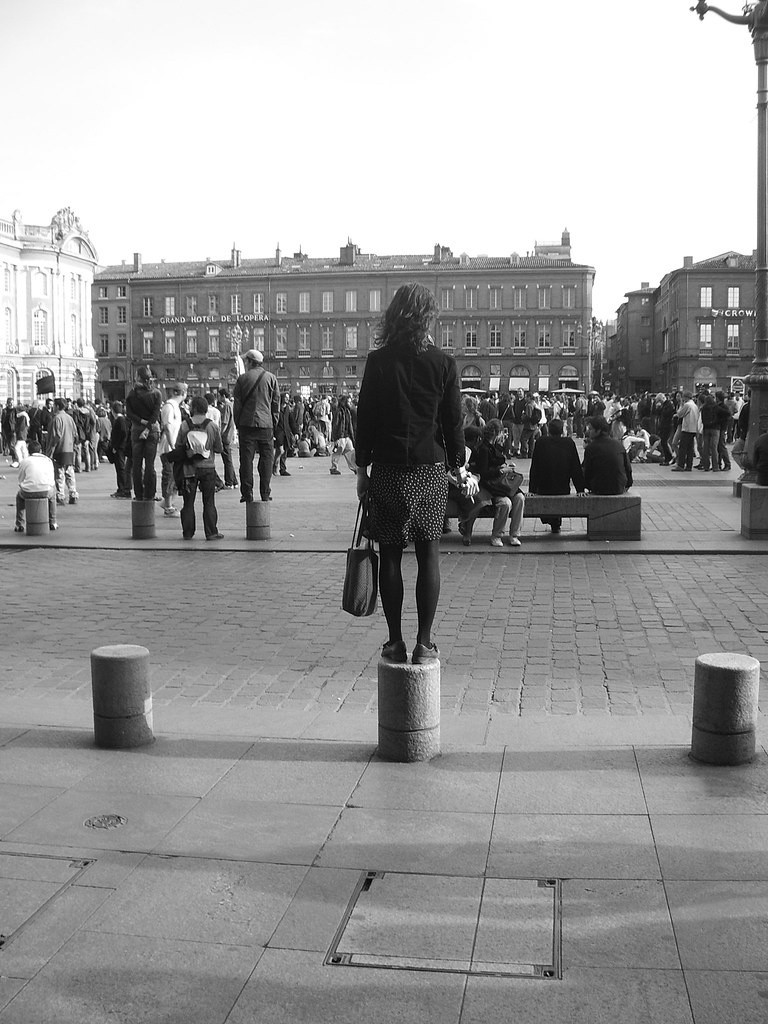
[241,349,262,363]
[172,382,188,392]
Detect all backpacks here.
[556,402,569,420]
[313,401,326,417]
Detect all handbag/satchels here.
[524,403,542,425]
[485,470,523,497]
[342,497,379,617]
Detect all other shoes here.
[329,469,341,474]
[462,535,471,546]
[10,461,19,467]
[14,526,24,532]
[56,455,163,505]
[206,532,225,541]
[50,523,59,530]
[354,469,357,474]
[163,506,181,518]
[630,453,731,472]
[444,527,452,533]
[215,470,291,503]
[380,641,408,662]
[183,536,192,540]
[503,446,534,460]
[411,641,440,663]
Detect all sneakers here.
[489,535,503,547]
[509,536,521,546]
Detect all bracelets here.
[457,472,472,488]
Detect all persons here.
[525,419,590,532]
[175,397,224,540]
[205,388,239,493]
[158,383,191,517]
[15,442,59,531]
[582,416,633,495]
[126,367,162,501]
[475,419,525,547]
[461,387,751,472]
[443,427,492,545]
[273,392,359,476]
[0,398,132,504]
[354,284,480,663]
[233,350,280,502]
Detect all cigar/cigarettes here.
[471,496,475,504]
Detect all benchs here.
[444,491,642,541]
[740,483,768,540]
